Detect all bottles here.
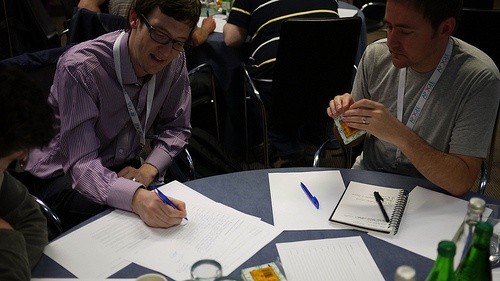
[424,240,458,281]
[190,259,222,281]
[452,221,492,281]
[450,197,486,272]
[394,265,416,281]
[135,273,168,281]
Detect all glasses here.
[16,151,30,174]
[141,13,192,52]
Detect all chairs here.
[0,0,366,236]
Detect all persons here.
[325,0,500,198]
[77,0,217,99]
[20,0,202,231]
[221,0,342,169]
[0,73,59,281]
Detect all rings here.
[362,117,368,124]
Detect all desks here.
[184,0,365,140]
[36,168,499,281]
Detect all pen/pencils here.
[207,10,209,17]
[301,182,319,209]
[374,192,389,222]
[152,184,188,221]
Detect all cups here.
[482,204,500,269]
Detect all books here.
[328,179,409,236]
